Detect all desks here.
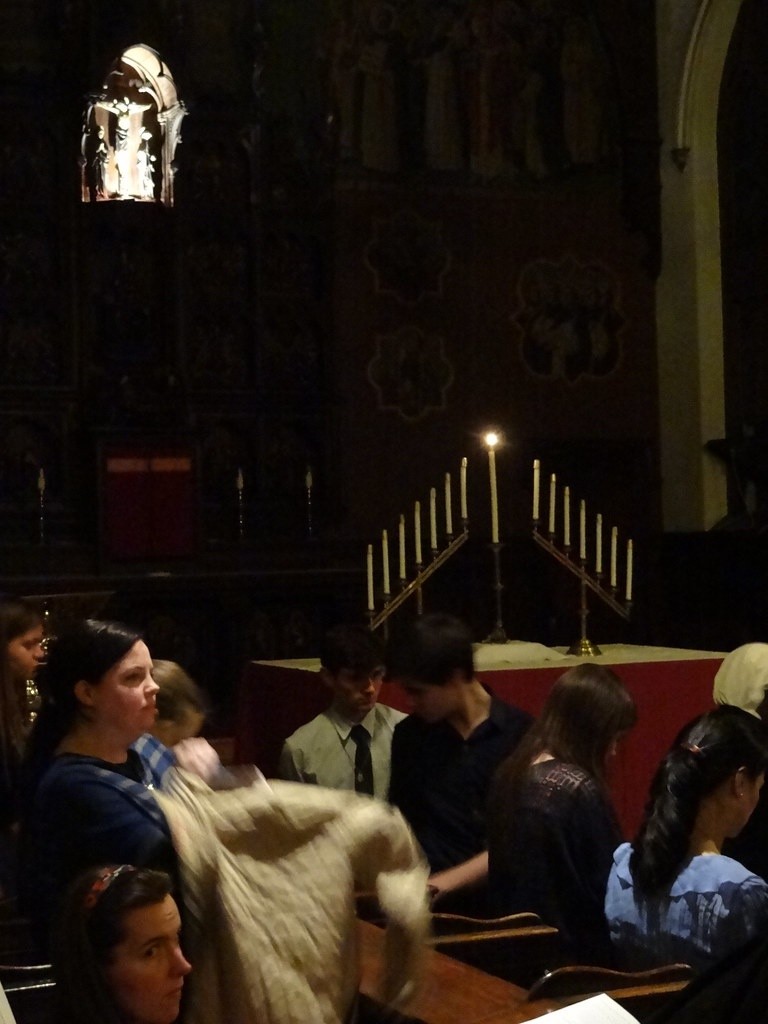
[233,642,728,843]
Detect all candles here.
[611,526,617,587]
[460,457,468,519]
[595,514,602,572]
[563,486,570,546]
[414,501,423,565]
[625,539,633,600]
[445,472,452,535]
[532,460,540,519]
[367,545,374,610]
[488,442,499,543]
[398,515,405,580]
[381,529,390,593]
[549,473,557,533]
[430,488,437,549]
[579,500,586,559]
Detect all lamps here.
[236,470,247,565]
[38,467,48,575]
[306,466,313,561]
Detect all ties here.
[351,724,373,796]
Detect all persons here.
[494,663,636,997]
[390,613,540,908]
[280,624,412,804]
[21,617,186,963]
[52,863,193,1024]
[606,703,768,1024]
[131,658,208,787]
[0,600,47,888]
